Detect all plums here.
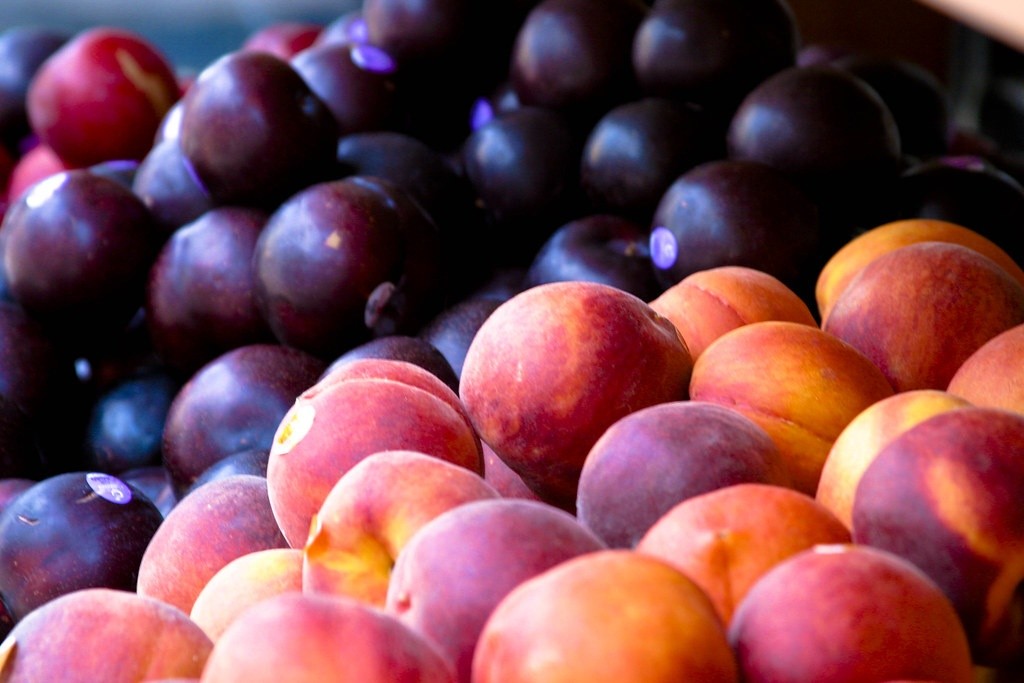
[0,0,1024,634]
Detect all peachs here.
[0,220,1023,683]
